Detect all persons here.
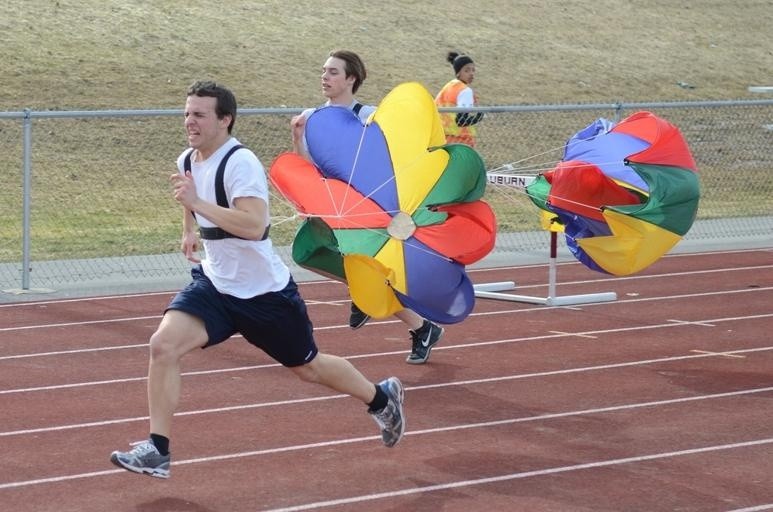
[434,51,491,154]
[109,80,407,483]
[288,50,446,367]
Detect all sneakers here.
[405,318,445,365]
[348,301,371,330]
[109,437,171,479]
[367,376,407,449]
